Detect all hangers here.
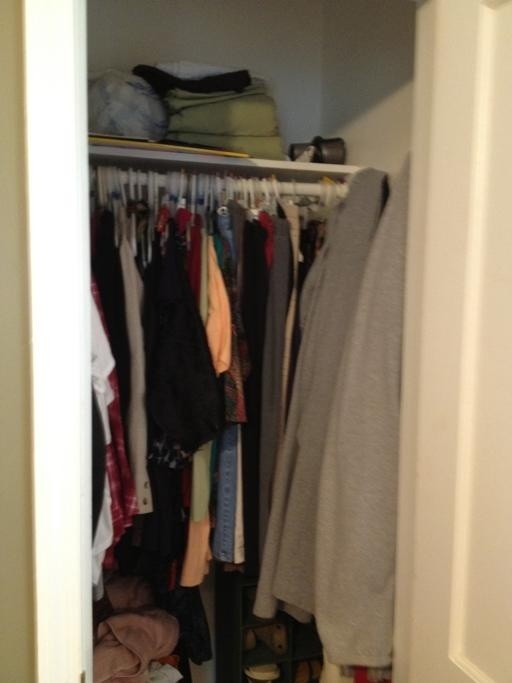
[87,164,346,268]
